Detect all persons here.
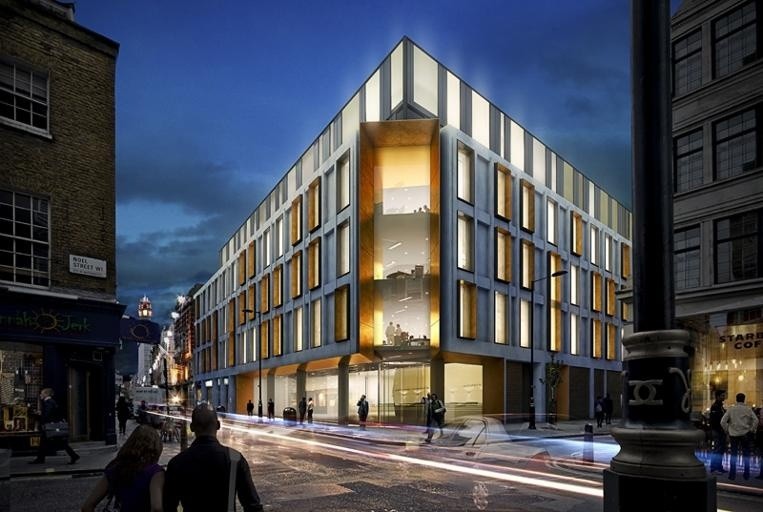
[29,388,79,465]
[357,395,369,428]
[81,424,166,512]
[422,391,446,443]
[707,390,763,480]
[299,396,307,423]
[382,321,401,346]
[127,398,164,424]
[307,398,314,423]
[595,395,605,428]
[268,398,275,418]
[604,392,613,425]
[247,400,254,414]
[116,396,130,434]
[163,402,264,511]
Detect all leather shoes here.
[28,457,46,465]
[67,454,80,464]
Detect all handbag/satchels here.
[42,421,70,439]
